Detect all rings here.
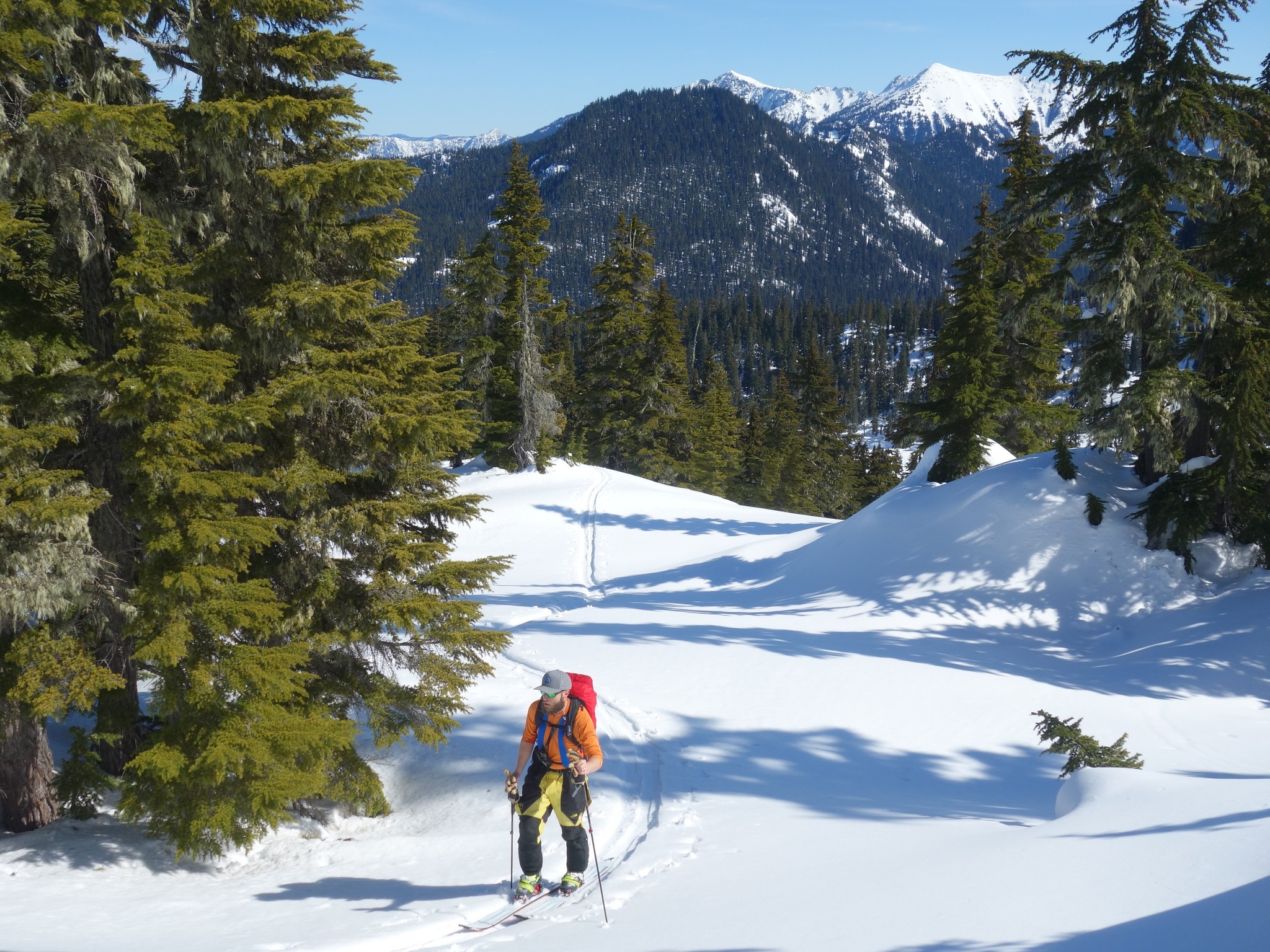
[583,768,585,771]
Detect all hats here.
[532,669,572,694]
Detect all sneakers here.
[516,871,541,897]
[561,869,584,892]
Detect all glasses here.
[540,690,566,697]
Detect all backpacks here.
[534,673,597,737]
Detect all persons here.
[505,669,603,902]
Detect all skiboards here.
[458,858,614,931]
